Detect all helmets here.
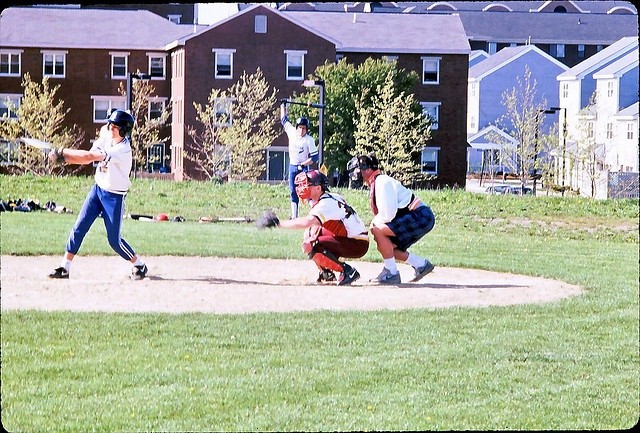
[296,118,308,131]
[347,156,377,190]
[294,170,330,207]
[107,111,134,131]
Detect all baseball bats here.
[201,218,250,223]
[281,99,326,108]
[20,137,62,165]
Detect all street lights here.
[303,80,325,171]
[532,107,560,197]
[125,73,152,144]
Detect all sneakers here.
[409,260,434,282]
[369,267,401,285]
[317,269,335,282]
[49,268,69,279]
[338,268,360,286]
[131,264,147,280]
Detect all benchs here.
[500,186,536,193]
[485,185,509,192]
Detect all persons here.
[344,154,434,286]
[91,105,116,149]
[47,110,150,283]
[276,98,321,216]
[274,168,369,288]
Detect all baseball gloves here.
[256,210,279,229]
